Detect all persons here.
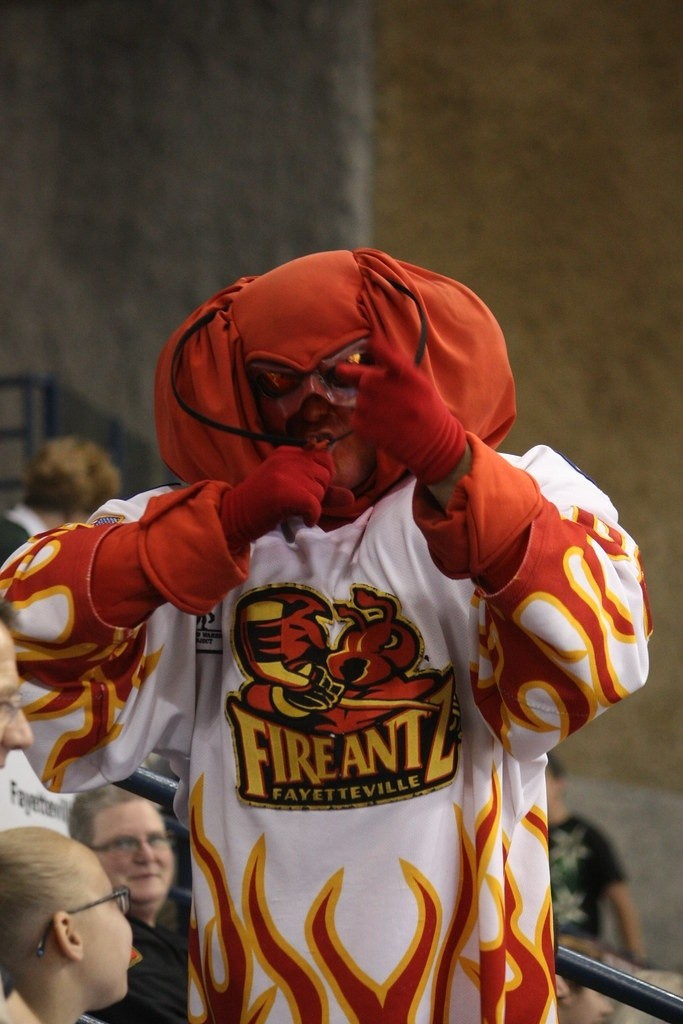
[1,436,120,573]
[545,754,647,967]
[0,599,33,780]
[68,781,192,1024]
[553,934,614,1024]
[0,247,651,1024]
[0,825,134,1024]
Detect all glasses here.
[238,338,372,403]
[37,886,130,956]
[84,832,175,860]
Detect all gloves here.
[335,334,466,487]
[221,445,354,552]
